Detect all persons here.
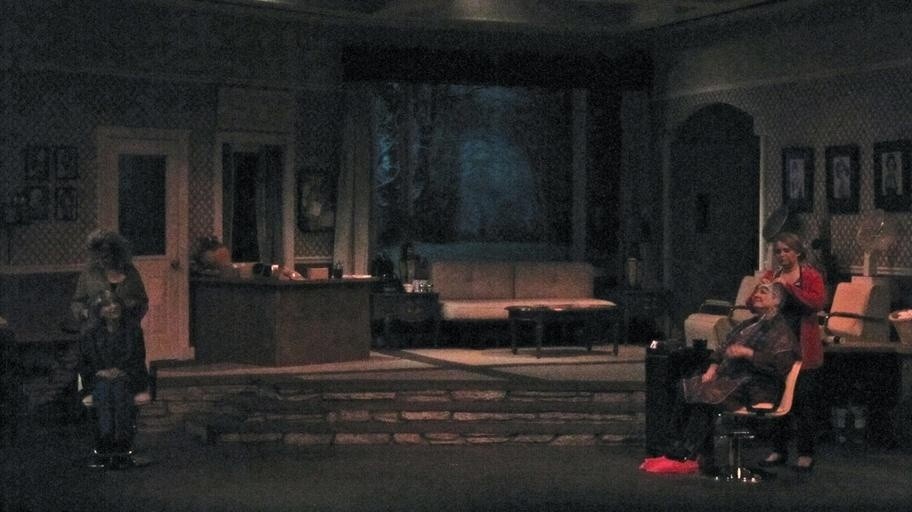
[73,229,149,393]
[756,231,828,477]
[883,155,899,196]
[649,278,799,460]
[835,159,851,198]
[82,290,148,453]
[790,161,801,199]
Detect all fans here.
[856,212,897,286]
[761,204,792,242]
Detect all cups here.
[404,284,414,292]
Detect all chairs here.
[817,281,893,345]
[684,271,768,351]
[715,358,803,483]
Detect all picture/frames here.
[873,139,912,211]
[26,143,80,220]
[825,143,858,216]
[298,166,336,233]
[781,146,814,213]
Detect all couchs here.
[412,240,618,322]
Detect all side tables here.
[372,291,441,349]
[601,287,673,345]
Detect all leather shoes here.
[794,455,815,472]
[87,446,113,468]
[759,451,785,467]
[119,448,150,467]
